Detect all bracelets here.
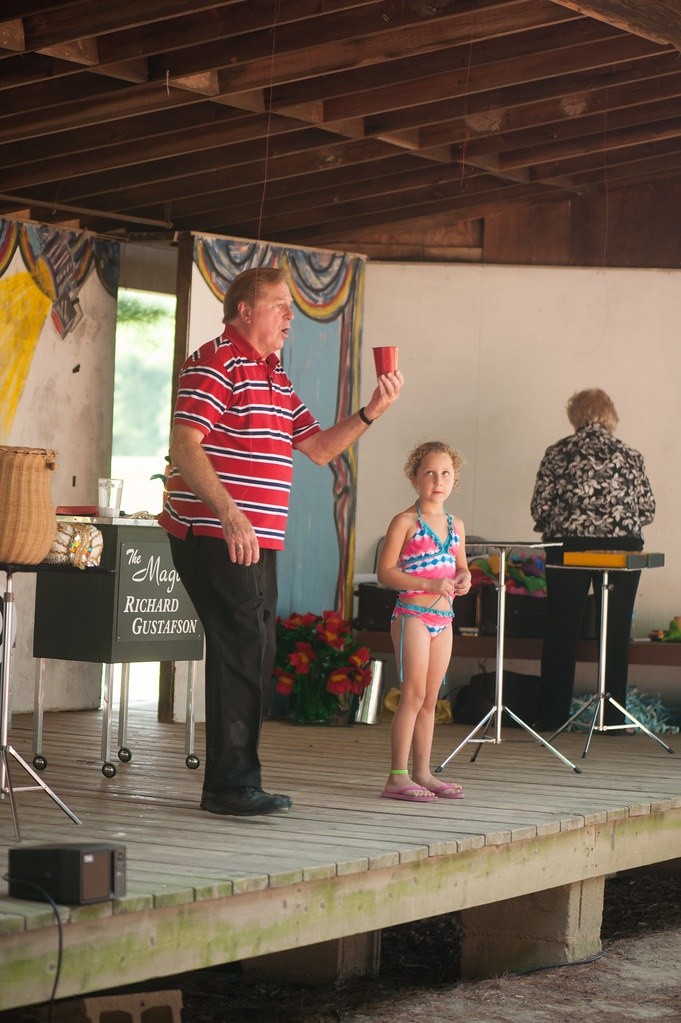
[359,406,374,426]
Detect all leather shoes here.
[200,780,292,816]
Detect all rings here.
[237,544,243,549]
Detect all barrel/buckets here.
[354,655,388,724]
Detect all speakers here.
[7,841,128,905]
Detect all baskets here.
[0,443,59,566]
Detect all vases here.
[297,691,354,726]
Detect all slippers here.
[429,780,465,798]
[382,786,438,802]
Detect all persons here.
[529,387,656,736]
[154,267,405,815]
[378,442,472,802]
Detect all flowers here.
[265,610,374,723]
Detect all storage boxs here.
[358,534,602,641]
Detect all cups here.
[97,477,123,517]
[374,346,400,379]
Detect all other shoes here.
[606,727,636,735]
[533,719,568,732]
